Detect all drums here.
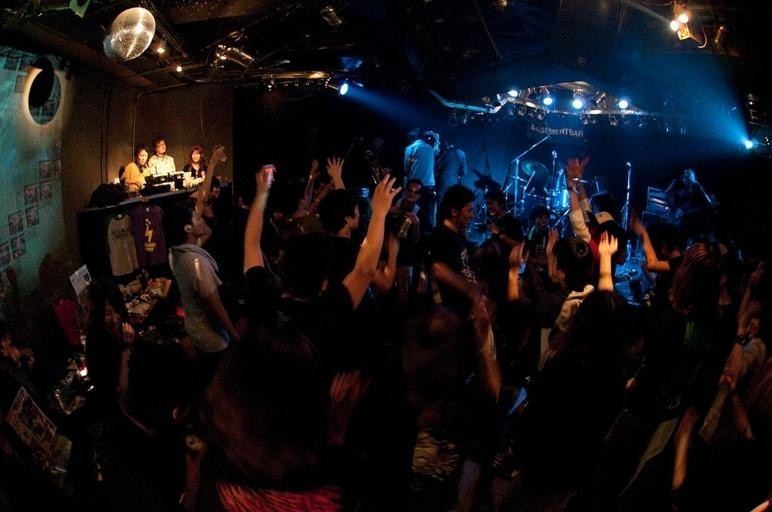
[528,210,570,239]
[546,189,571,208]
[522,193,551,219]
[503,175,527,196]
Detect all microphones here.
[552,150,559,159]
[626,162,631,169]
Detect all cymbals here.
[521,160,549,179]
[572,178,588,183]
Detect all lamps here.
[447,86,693,136]
[217,42,257,69]
[325,76,349,95]
